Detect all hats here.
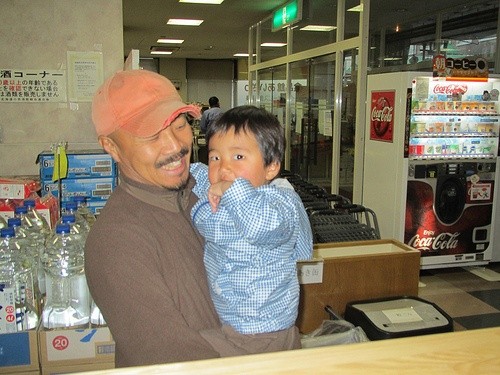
[92,69,202,140]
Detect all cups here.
[410,144,493,156]
[412,100,496,112]
[411,122,495,134]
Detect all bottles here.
[42,196,107,329]
[0,198,49,331]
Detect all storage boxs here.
[0,147,125,375]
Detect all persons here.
[188,106,313,356]
[85,70,224,366]
[200,96,224,131]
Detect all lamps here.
[377,55,405,61]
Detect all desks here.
[58,326,500,375]
